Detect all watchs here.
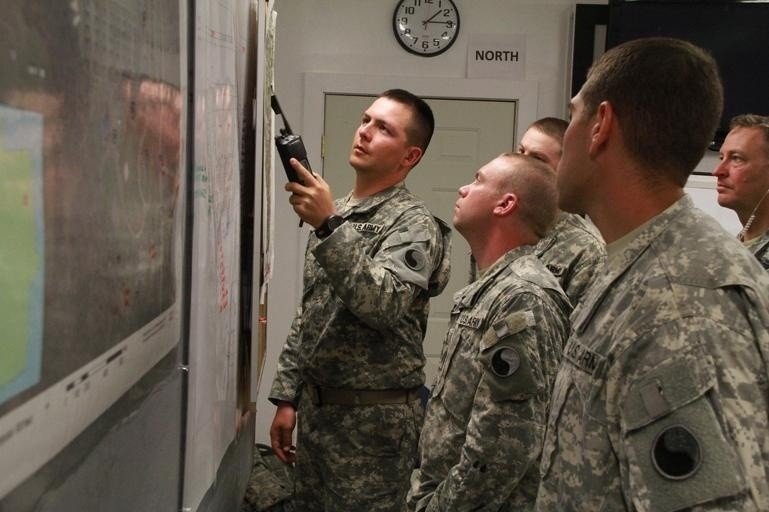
[310,215,347,237]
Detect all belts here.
[309,385,421,404]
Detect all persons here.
[520,118,603,307]
[555,38,768,510]
[416,153,576,511]
[268,89,451,512]
[712,112,768,271]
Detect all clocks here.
[393,0,460,57]
